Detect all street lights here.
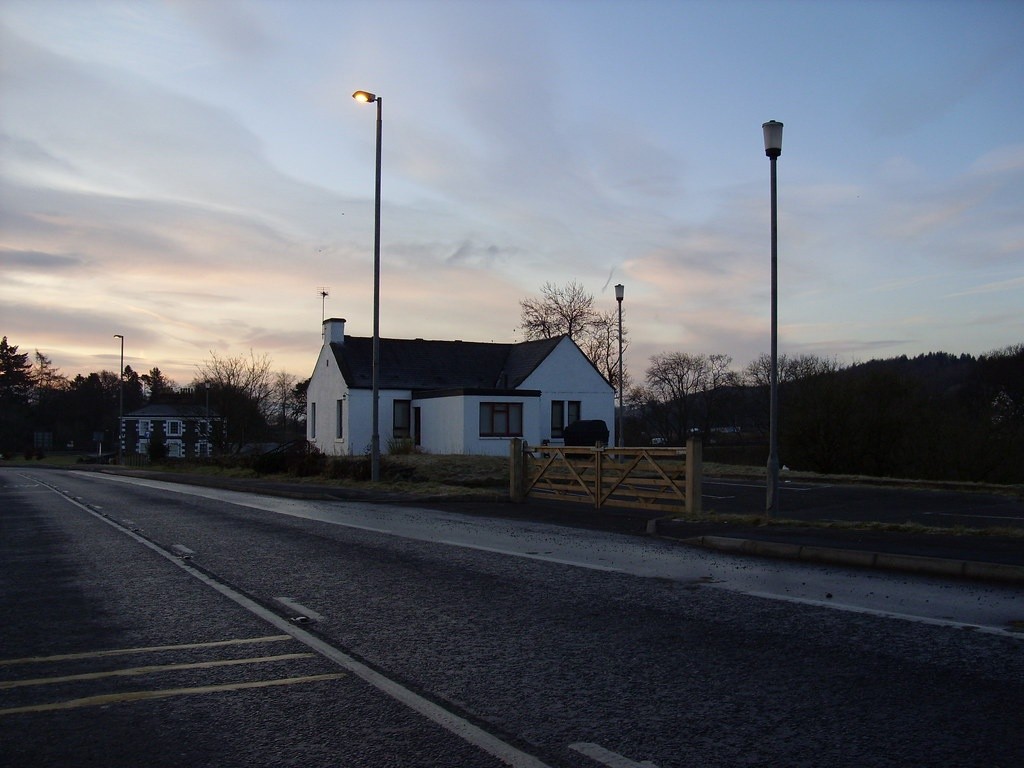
[761,119,786,515]
[614,284,625,462]
[113,334,125,465]
[352,91,389,485]
